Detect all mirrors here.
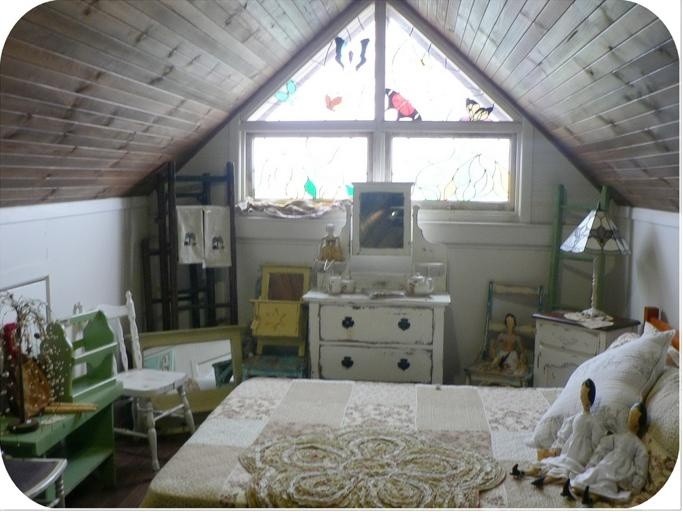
[124,324,250,413]
[353,183,411,256]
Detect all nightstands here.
[531,309,641,388]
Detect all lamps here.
[559,200,631,321]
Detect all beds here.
[148,307,679,508]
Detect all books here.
[531,310,578,324]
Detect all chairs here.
[75,289,195,472]
[0,447,68,508]
[464,280,545,387]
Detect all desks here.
[0,380,123,508]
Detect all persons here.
[559,400,650,506]
[487,313,528,375]
[509,377,604,489]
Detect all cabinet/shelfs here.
[301,287,451,384]
[248,263,312,358]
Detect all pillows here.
[525,321,679,497]
[600,330,679,461]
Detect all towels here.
[202,206,232,269]
[176,205,204,265]
